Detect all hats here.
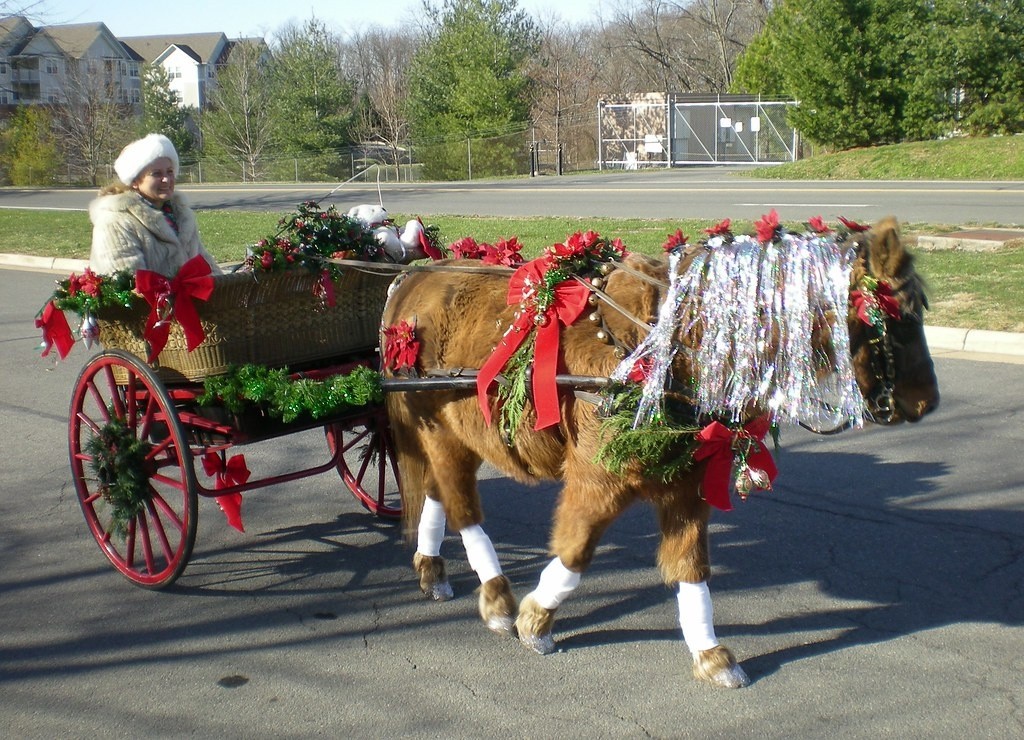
[114,133,180,187]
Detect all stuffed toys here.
[349,205,424,255]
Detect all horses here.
[379,216,940,688]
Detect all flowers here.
[244,203,385,283]
[69,266,103,299]
[378,209,903,382]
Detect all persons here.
[89,134,225,276]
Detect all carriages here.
[65,203,940,688]
